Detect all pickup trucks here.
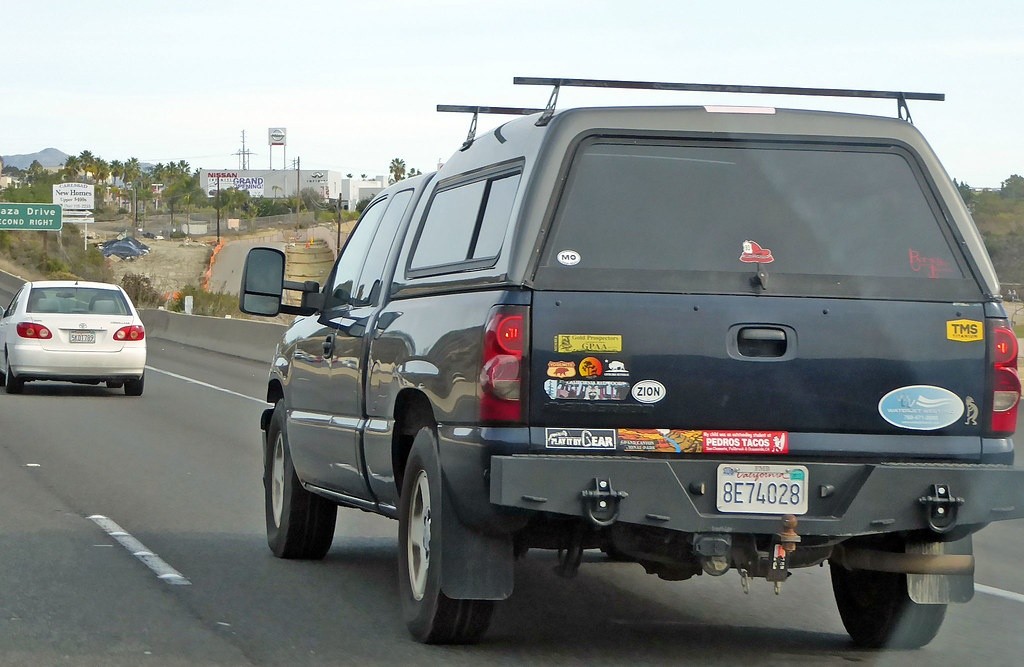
[237,75,1024,653]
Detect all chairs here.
[37,298,61,312]
[93,300,116,313]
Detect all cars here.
[0,279,148,397]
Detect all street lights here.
[151,184,164,209]
